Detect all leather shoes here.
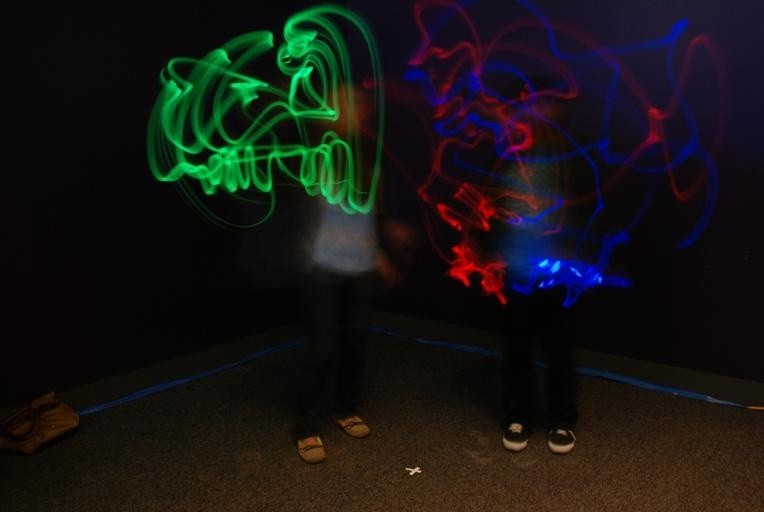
[337,416,369,437]
[298,436,325,463]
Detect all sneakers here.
[502,423,529,451]
[548,430,576,453]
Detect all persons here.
[493,70,579,457]
[289,65,419,465]
